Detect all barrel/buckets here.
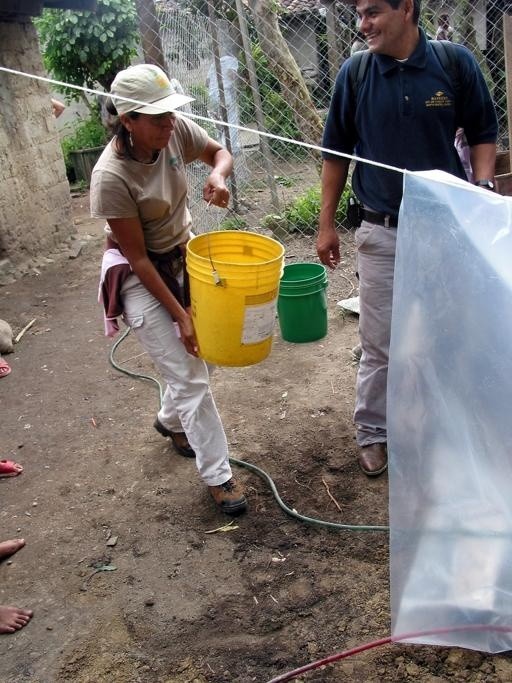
[277,264,330,344]
[186,197,285,368]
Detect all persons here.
[205,43,243,157]
[314,0,500,476]
[351,30,368,56]
[87,63,250,513]
[50,97,66,118]
[433,13,450,40]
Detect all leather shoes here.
[356,440,385,475]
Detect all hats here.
[109,63,197,119]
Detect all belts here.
[362,210,396,227]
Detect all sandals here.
[2,357,11,378]
[3,458,23,478]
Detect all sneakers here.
[150,420,193,453]
[208,477,247,516]
